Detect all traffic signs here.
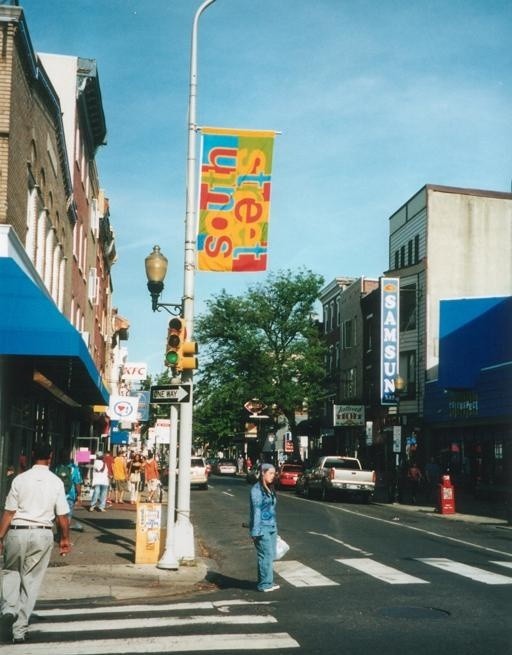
[147,383,193,406]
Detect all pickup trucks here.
[294,454,379,504]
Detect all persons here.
[237,452,262,474]
[16,447,169,542]
[1,442,72,643]
[247,462,281,595]
[400,455,480,506]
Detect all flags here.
[194,127,275,273]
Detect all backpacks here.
[55,462,76,495]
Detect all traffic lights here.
[182,338,201,373]
[163,315,187,371]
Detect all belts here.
[8,524,53,531]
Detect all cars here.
[153,456,238,490]
[246,462,279,486]
[274,462,305,490]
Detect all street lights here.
[143,240,200,562]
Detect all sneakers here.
[114,499,125,504]
[257,582,282,593]
[88,505,96,513]
[0,612,30,644]
[97,507,107,512]
